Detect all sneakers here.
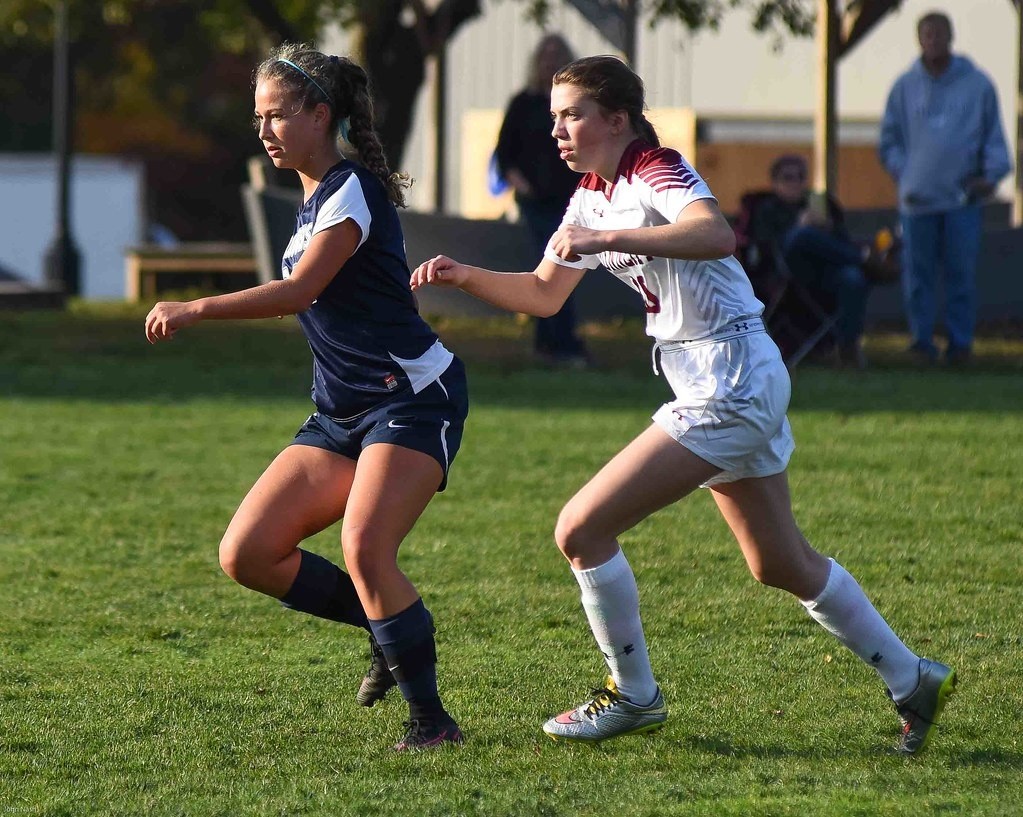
[356,635,398,708]
[541,675,668,746]
[392,715,464,755]
[885,657,959,756]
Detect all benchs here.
[124,242,258,304]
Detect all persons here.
[497,34,596,359]
[145,41,472,752]
[409,57,959,758]
[881,12,1013,364]
[735,154,864,361]
